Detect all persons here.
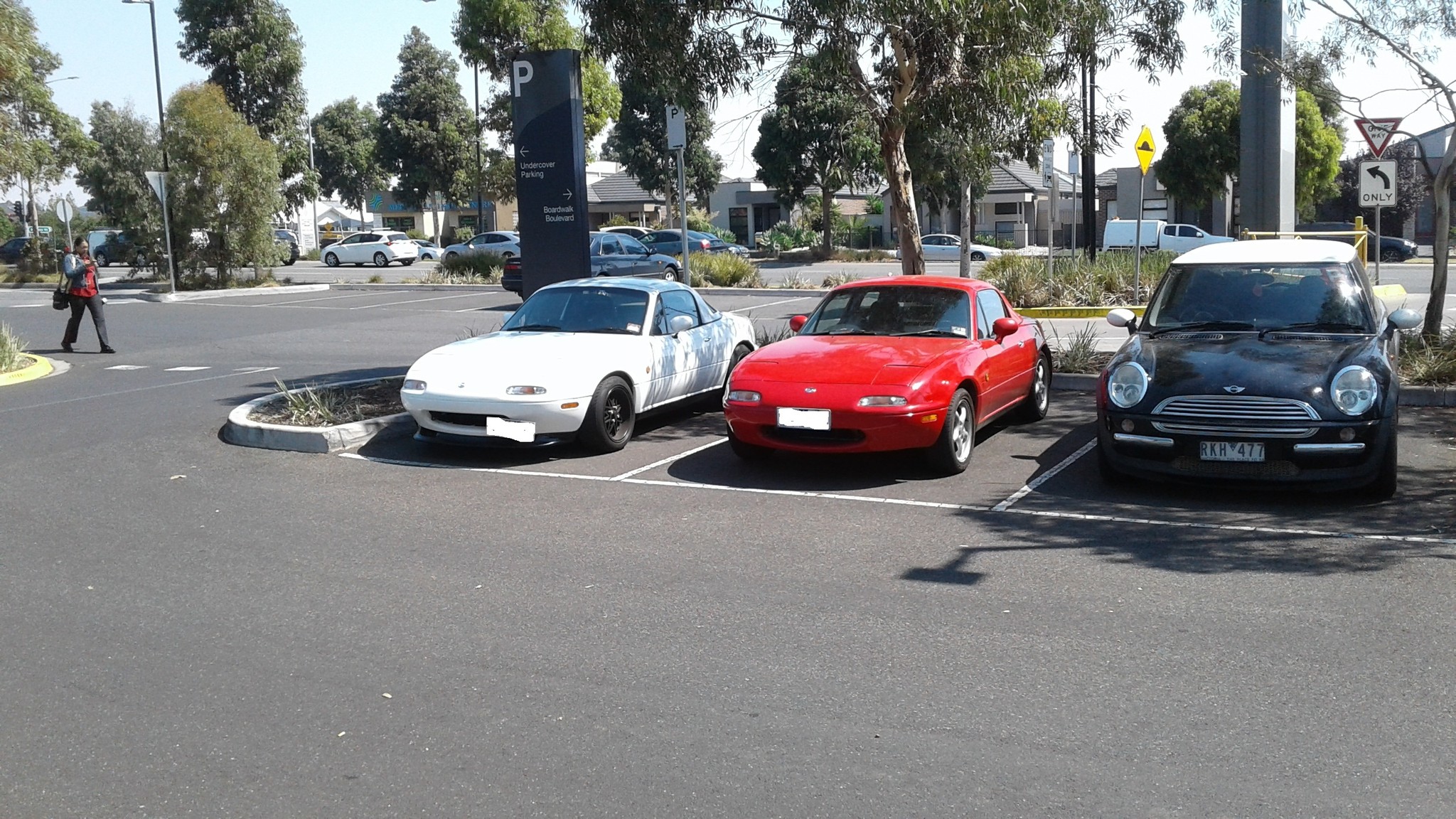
[61,236,116,353]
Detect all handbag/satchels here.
[52,287,70,310]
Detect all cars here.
[440,230,521,270]
[598,226,655,242]
[0,237,71,265]
[1095,237,1424,502]
[410,239,446,261]
[93,228,140,268]
[320,227,419,267]
[633,229,751,263]
[896,234,1004,262]
[501,231,686,301]
[124,244,153,268]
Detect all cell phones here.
[90,260,97,263]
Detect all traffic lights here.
[26,215,31,222]
[13,201,21,216]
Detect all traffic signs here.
[1359,160,1398,207]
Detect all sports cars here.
[399,275,762,455]
[721,274,1053,476]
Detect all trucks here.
[1098,216,1240,254]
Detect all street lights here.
[16,76,82,237]
[122,0,181,282]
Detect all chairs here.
[942,239,947,244]
[1274,276,1354,325]
[1177,266,1241,322]
[566,294,621,329]
[657,295,695,334]
[866,301,906,332]
[934,299,969,334]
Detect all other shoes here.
[61,340,73,352]
[100,345,116,353]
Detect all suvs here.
[1294,220,1418,264]
[269,229,301,266]
